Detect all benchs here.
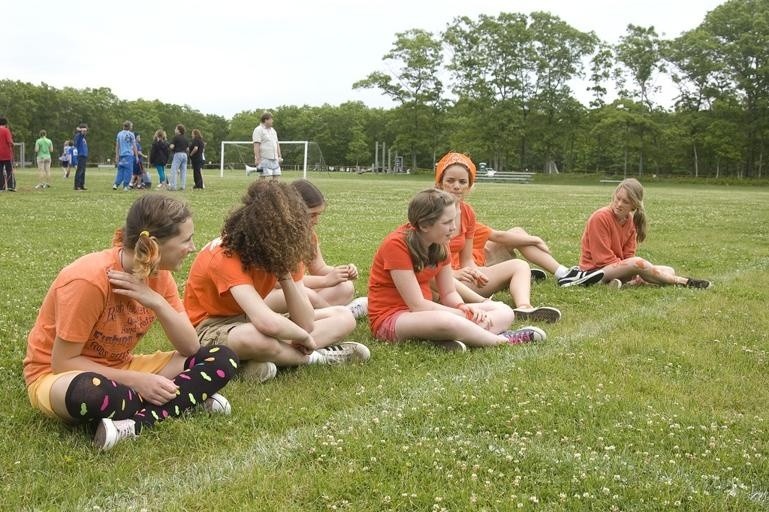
[472,168,534,185]
[600,174,623,185]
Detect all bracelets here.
[456,302,465,308]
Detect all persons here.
[20,194,239,449]
[367,189,548,354]
[264,179,369,320]
[471,219,604,288]
[184,181,370,383]
[435,153,562,326]
[0,113,284,186]
[578,177,709,289]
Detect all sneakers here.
[318,342,370,367]
[503,327,546,345]
[347,297,368,318]
[93,418,135,450]
[514,305,561,322]
[559,266,604,287]
[531,268,547,281]
[202,393,231,414]
[609,278,622,288]
[245,361,276,382]
[437,340,466,354]
[684,278,712,289]
